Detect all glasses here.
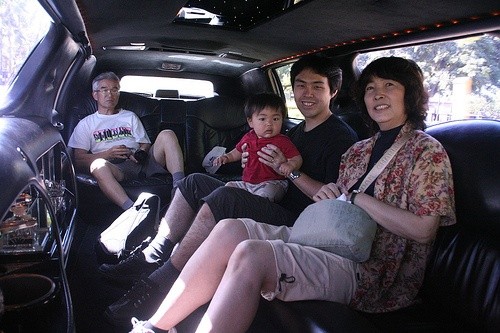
[94,88,120,96]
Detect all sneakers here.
[96,248,158,290]
[104,273,161,328]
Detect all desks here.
[0,140,79,333]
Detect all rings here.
[271,159,274,162]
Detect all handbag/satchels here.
[288,200,378,262]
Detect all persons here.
[213,93,302,202]
[67,72,184,210]
[130,55,457,333]
[96,52,360,327]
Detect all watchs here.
[350,190,360,204]
[290,169,301,180]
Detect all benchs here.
[261,112,500,333]
[66,90,250,204]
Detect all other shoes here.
[128,317,178,333]
